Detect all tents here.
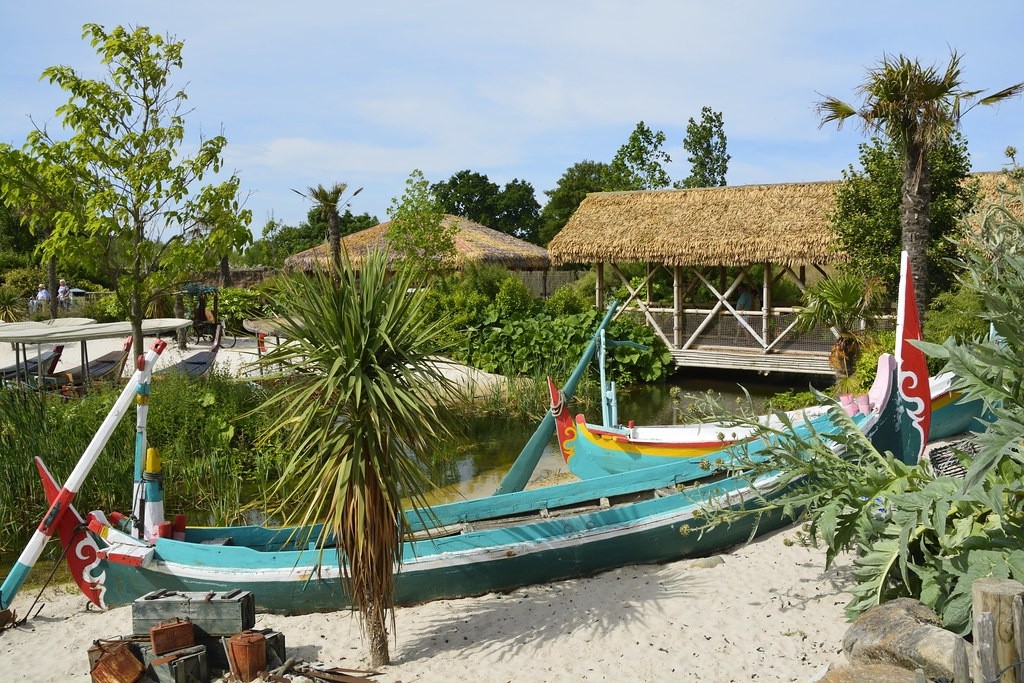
[70,288,86,297]
[171,282,219,310]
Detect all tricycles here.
[185,285,236,350]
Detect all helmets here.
[36,283,45,289]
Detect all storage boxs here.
[87,587,287,683]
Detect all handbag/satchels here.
[62,297,71,302]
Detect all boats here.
[547,353,1001,483]
[33,335,133,394]
[33,253,932,613]
[150,325,223,384]
[0,345,64,383]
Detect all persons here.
[28,283,50,314]
[56,279,71,312]
[195,294,216,342]
[733,285,760,346]
